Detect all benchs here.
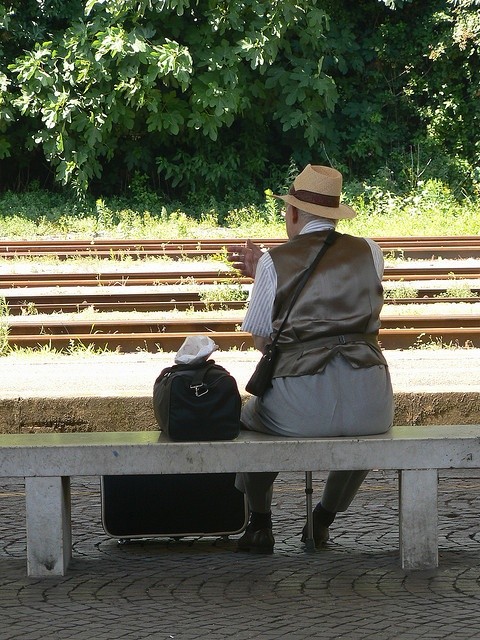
[0,425,480,576]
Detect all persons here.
[224,161,395,550]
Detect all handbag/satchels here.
[154,359,241,442]
[245,344,278,395]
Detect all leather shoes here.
[238,529,274,553]
[300,521,328,545]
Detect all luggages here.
[100,472,248,543]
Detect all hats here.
[268,164,356,219]
